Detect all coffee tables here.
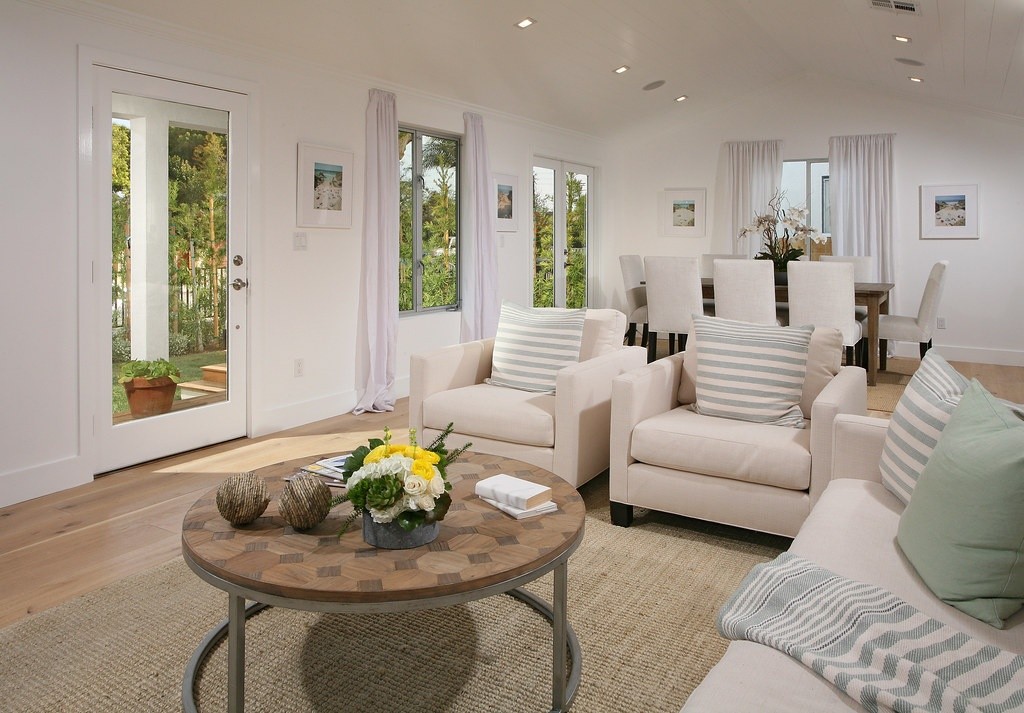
[181,448,585,713]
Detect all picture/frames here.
[921,184,980,239]
[296,142,355,229]
[664,190,706,236]
[491,172,519,232]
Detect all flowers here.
[737,186,828,271]
[328,422,471,544]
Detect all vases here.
[363,506,439,549]
[773,269,789,285]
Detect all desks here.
[640,278,895,386]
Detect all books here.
[283,453,355,487]
[475,474,559,519]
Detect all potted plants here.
[117,356,183,418]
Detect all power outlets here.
[936,316,947,329]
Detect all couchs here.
[609,318,868,543]
[679,347,1024,713]
[409,299,647,490]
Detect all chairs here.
[861,262,944,375]
[702,253,747,279]
[788,260,863,365]
[619,254,684,356]
[644,256,714,365]
[712,258,788,327]
[819,255,874,367]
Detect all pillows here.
[896,376,1024,631]
[480,299,588,397]
[690,313,816,429]
[878,348,1024,507]
